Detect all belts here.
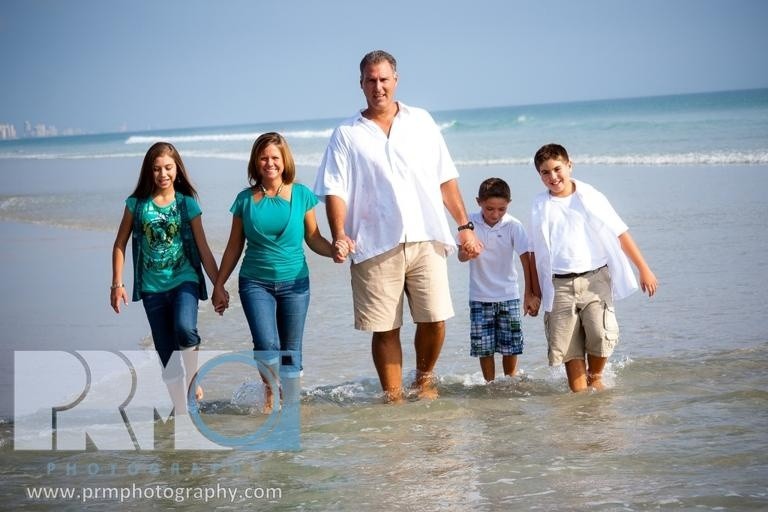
[551,265,609,278]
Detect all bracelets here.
[111,283,124,289]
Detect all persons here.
[455,177,541,380]
[530,143,658,392]
[210,131,351,411]
[315,50,483,404]
[108,141,227,415]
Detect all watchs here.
[458,222,473,230]
